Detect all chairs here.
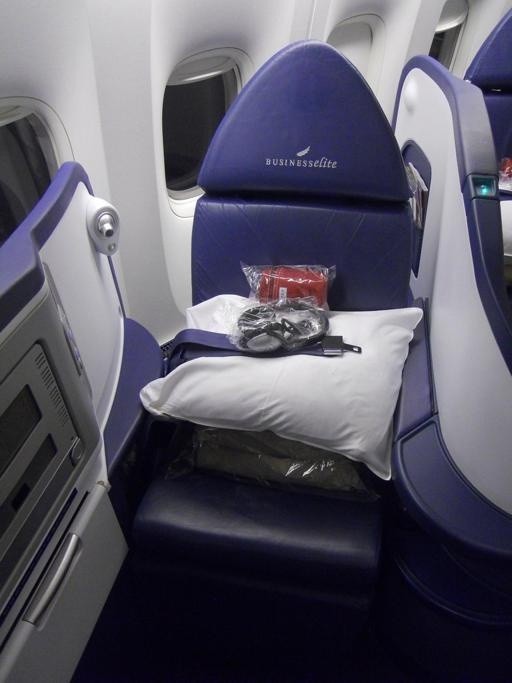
[122,6,512,683]
